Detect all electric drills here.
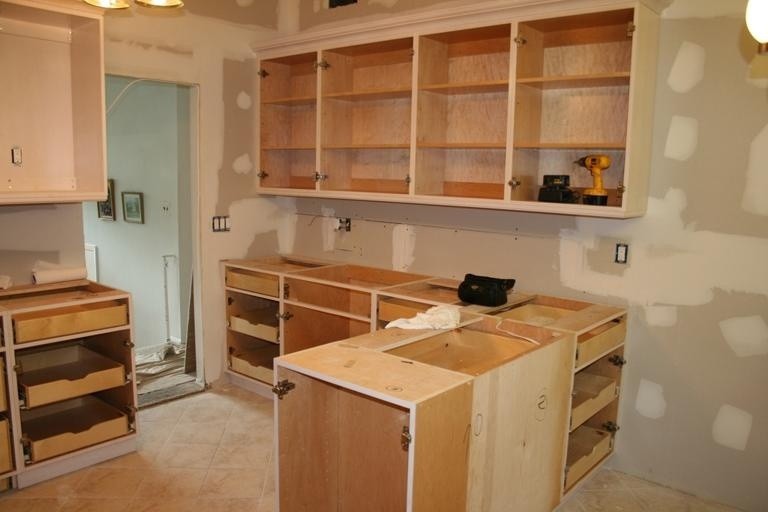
[573,155,611,205]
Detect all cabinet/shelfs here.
[250,0,662,216]
[0,0,109,204]
[220,265,627,512]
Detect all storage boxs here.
[0,279,138,494]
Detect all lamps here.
[85,0,184,10]
[745,0,768,54]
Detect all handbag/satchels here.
[459,274,515,306]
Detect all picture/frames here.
[96,180,115,221]
[121,191,144,224]
